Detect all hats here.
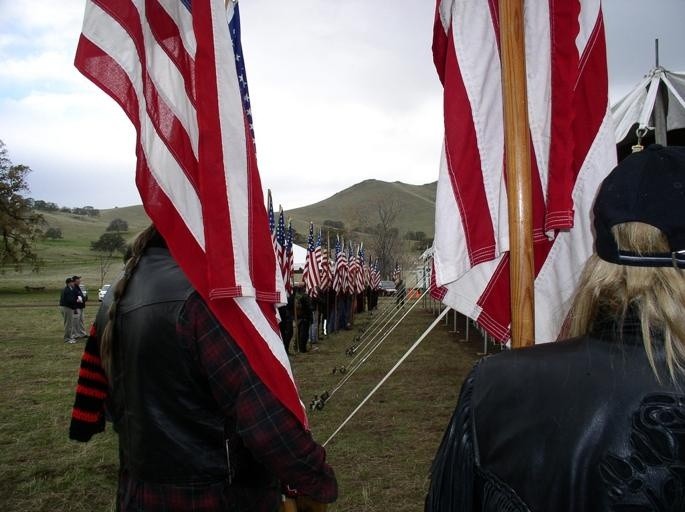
[592,142,685,268]
[66,275,81,283]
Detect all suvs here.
[379,279,398,296]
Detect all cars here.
[97,284,111,301]
[79,284,89,301]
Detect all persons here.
[69,242,132,442]
[71,276,89,338]
[395,280,406,309]
[59,278,78,344]
[425,145,682,510]
[99,219,338,511]
[276,286,378,355]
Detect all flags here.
[430,0,618,350]
[267,194,382,294]
[75,0,306,431]
[393,262,400,282]
